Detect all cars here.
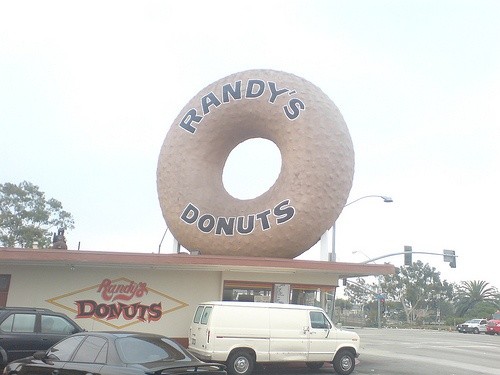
[1,331,229,375]
[456,320,471,333]
[462,319,488,334]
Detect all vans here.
[186,301,361,375]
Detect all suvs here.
[0,306,88,375]
[485,312,500,335]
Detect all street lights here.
[328,194,393,318]
[351,250,383,329]
